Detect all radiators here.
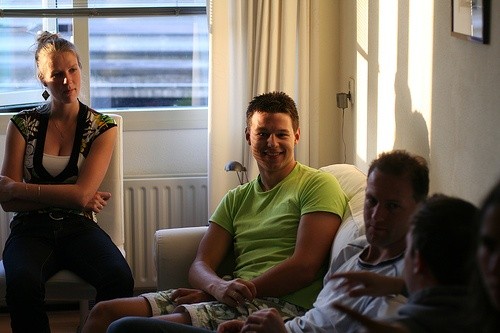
[123,177,208,287]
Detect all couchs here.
[153,163,369,290]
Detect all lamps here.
[225,161,247,185]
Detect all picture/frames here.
[450,0,491,44]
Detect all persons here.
[82,91,350,333]
[105,150,430,333]
[330,179,500,333]
[0,30,134,333]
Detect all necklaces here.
[50,114,76,137]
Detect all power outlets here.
[348,79,355,104]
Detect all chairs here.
[0,112,126,333]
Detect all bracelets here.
[24,183,29,210]
[37,184,42,211]
